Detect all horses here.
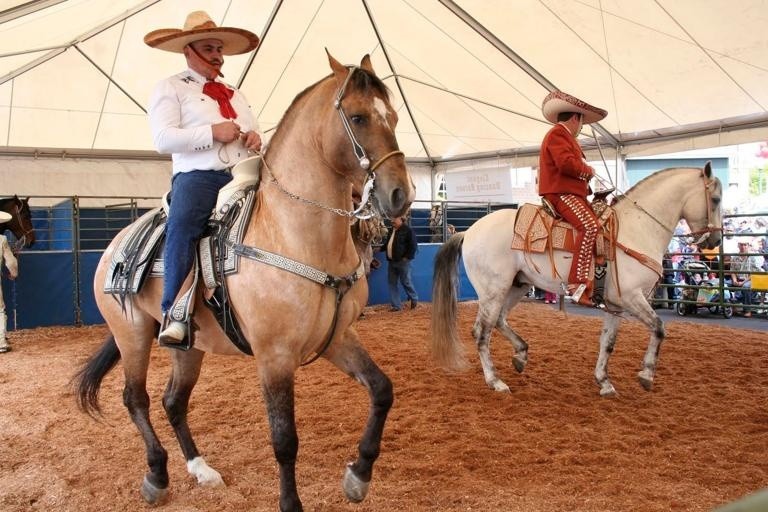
[431,160,722,400]
[0,194,35,248]
[67,46,418,512]
[428,204,456,243]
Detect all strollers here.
[676,259,732,319]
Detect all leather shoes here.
[160,322,189,344]
[0,337,12,353]
[567,282,592,306]
[743,311,752,318]
[411,299,417,308]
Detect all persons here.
[374,215,418,312]
[143,12,261,346]
[652,208,768,316]
[0,211,18,354]
[534,288,556,304]
[360,244,373,319]
[447,224,457,240]
[539,112,601,306]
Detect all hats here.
[541,91,608,125]
[143,11,259,55]
[0,209,13,224]
